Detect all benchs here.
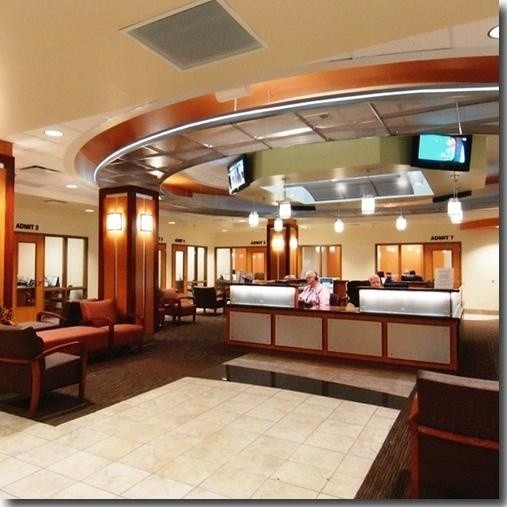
[5,309,62,333]
[33,326,110,360]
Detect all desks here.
[14,282,61,305]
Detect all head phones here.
[314,272,318,280]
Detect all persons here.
[368,271,384,288]
[298,270,331,307]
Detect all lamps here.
[276,175,293,221]
[447,170,462,216]
[395,198,406,231]
[138,212,154,233]
[105,210,124,232]
[450,184,463,226]
[359,169,376,215]
[273,206,283,232]
[334,207,343,235]
[245,191,260,227]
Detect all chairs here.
[159,286,196,324]
[0,314,91,418]
[34,298,96,326]
[79,296,148,357]
[404,364,498,498]
[191,285,226,315]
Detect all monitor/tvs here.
[46,277,59,287]
[319,278,333,284]
[410,133,473,172]
[227,153,250,196]
